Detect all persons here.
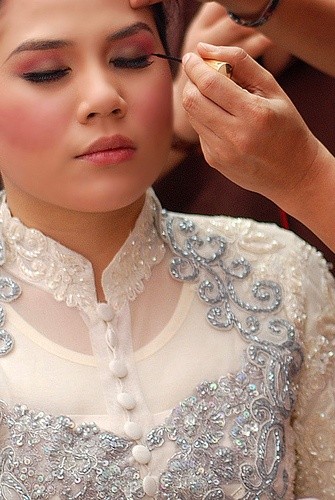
[130,0,335,277]
[0,0,335,500]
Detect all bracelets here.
[172,132,201,152]
[227,0,278,29]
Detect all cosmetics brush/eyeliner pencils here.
[152,54,233,78]
